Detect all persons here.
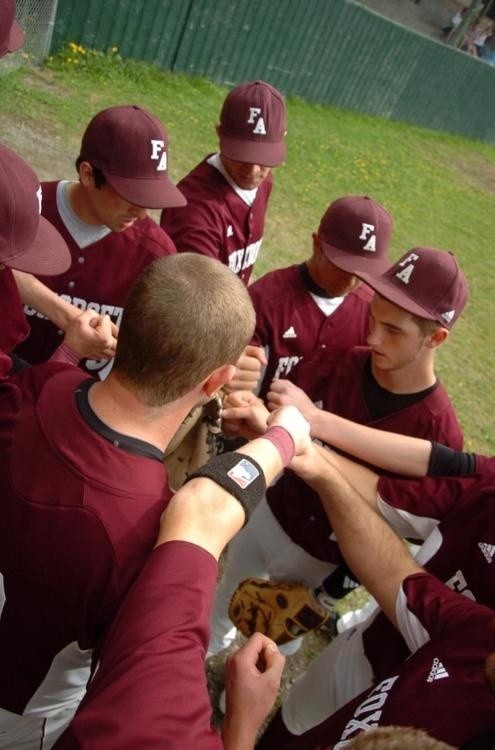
[439,7,495,59]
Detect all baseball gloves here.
[227,578,332,648]
[163,393,221,491]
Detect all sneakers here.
[317,610,342,641]
[216,687,228,715]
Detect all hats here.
[354,247,469,331]
[0,142,72,280]
[221,80,286,168]
[80,105,188,208]
[319,194,395,276]
